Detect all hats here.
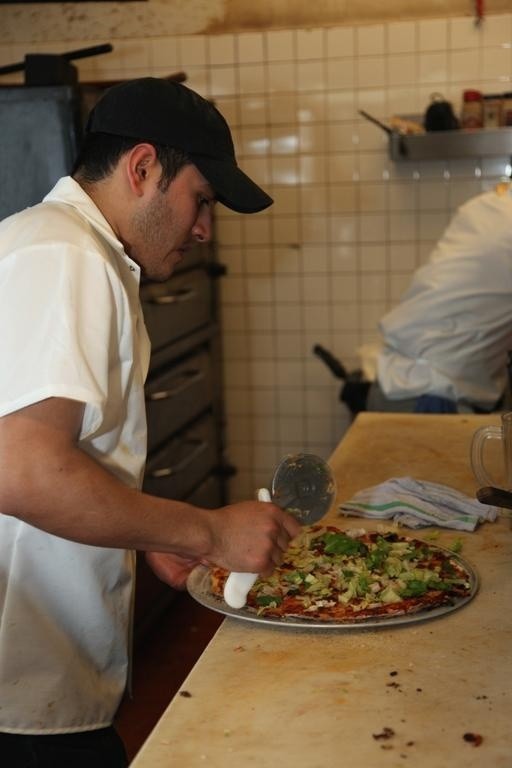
[85,75,273,213]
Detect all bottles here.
[460,90,511,128]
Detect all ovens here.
[3,79,236,612]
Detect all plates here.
[187,575,484,631]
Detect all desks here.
[129,412,512,768]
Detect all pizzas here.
[209,526,471,621]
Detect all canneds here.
[462,90,511,130]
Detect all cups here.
[469,412,511,495]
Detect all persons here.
[0,75,305,767]
[362,180,511,414]
